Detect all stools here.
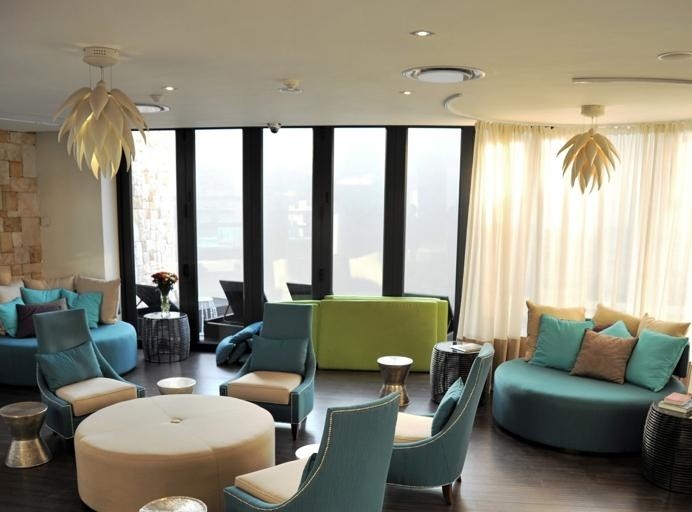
[157,377,197,393]
[0,401,54,469]
[640,400,692,494]
[430,340,488,409]
[377,355,414,407]
[137,495,208,512]
[295,442,321,459]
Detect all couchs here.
[492,344,692,465]
[0,319,138,386]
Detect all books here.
[664,392,691,405]
[452,343,482,352]
[658,398,692,413]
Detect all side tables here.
[141,311,190,364]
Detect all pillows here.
[524,300,690,392]
[215,322,263,366]
[299,453,319,490]
[430,377,465,436]
[33,340,104,391]
[250,334,310,377]
[0,272,122,339]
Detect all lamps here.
[556,103,622,194]
[51,47,149,180]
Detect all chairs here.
[31,308,145,456]
[386,341,496,505]
[225,390,401,512]
[220,303,317,443]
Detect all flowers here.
[151,271,179,304]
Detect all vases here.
[160,295,171,317]
